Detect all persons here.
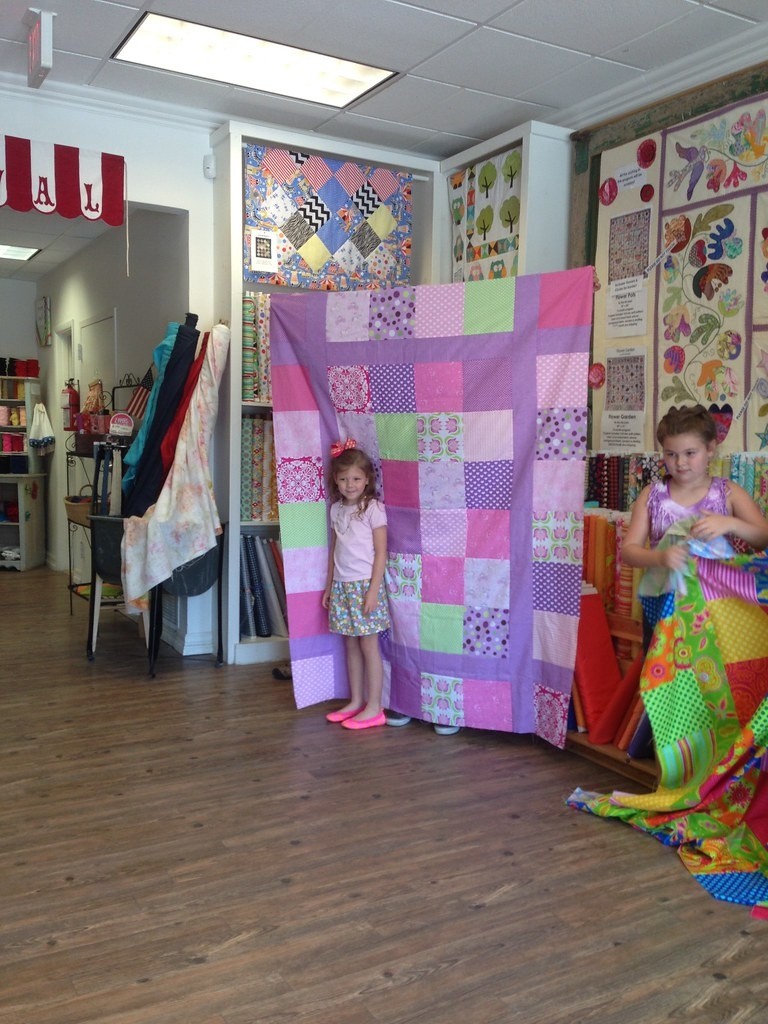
[386,265,601,736]
[621,407,768,656]
[322,448,389,730]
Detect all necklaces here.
[335,502,352,535]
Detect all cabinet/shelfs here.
[0,374,51,573]
[210,58,768,792]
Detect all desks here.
[84,512,231,678]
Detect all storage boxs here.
[62,488,110,526]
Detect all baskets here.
[63,485,110,526]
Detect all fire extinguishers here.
[60,379,79,431]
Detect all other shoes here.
[386,710,411,727]
[434,723,460,735]
[326,704,365,722]
[340,711,385,729]
